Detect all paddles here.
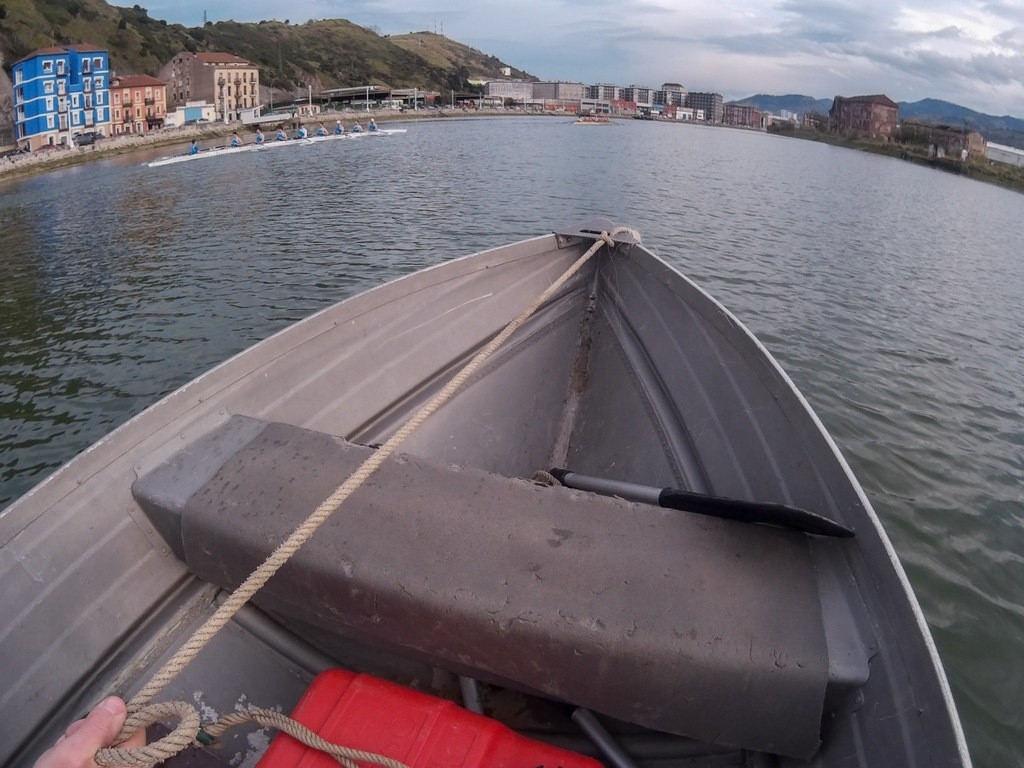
[544,696,641,768]
[546,465,859,541]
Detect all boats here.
[572,115,613,125]
[147,127,407,167]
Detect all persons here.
[257,128,265,145]
[335,120,344,135]
[315,122,327,136]
[275,124,287,141]
[296,123,307,138]
[190,139,199,155]
[353,120,363,133]
[368,118,377,132]
[232,132,242,146]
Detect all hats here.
[371,119,374,122]
[337,120,341,124]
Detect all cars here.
[186,117,211,127]
[34,144,55,152]
[73,133,104,145]
[0,148,29,157]
[380,103,451,110]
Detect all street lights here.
[366,87,369,112]
[308,84,314,117]
[451,89,455,111]
[413,87,417,111]
